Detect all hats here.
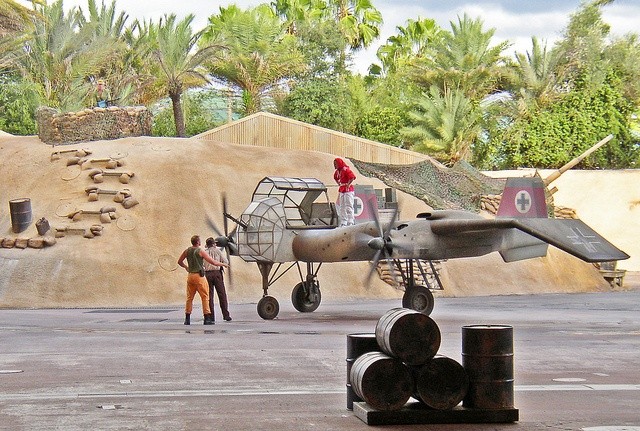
[205,236,218,247]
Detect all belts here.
[189,272,199,273]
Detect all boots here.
[184,313,190,325]
[204,314,215,325]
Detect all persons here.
[177,235,231,326]
[332,158,356,228]
[199,237,232,322]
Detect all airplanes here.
[204,176,630,319]
